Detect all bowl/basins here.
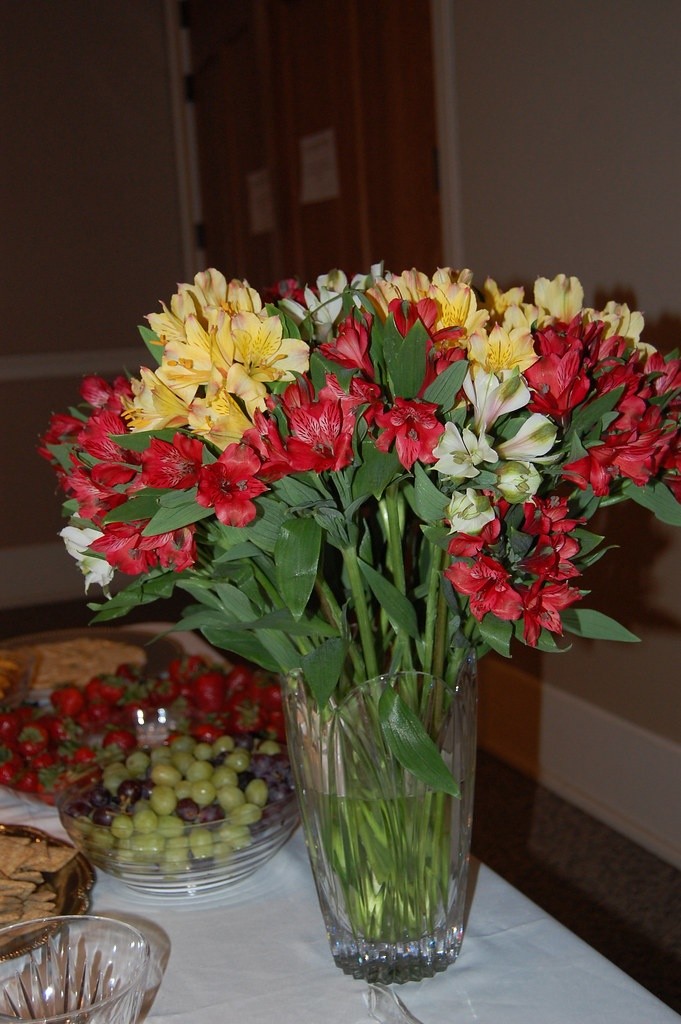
[54,747,300,897]
[0,915,151,1024]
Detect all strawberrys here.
[0,650,287,806]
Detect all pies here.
[0,639,147,690]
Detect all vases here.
[279,645,475,987]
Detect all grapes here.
[64,734,296,876]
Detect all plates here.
[0,823,96,961]
[0,688,184,809]
[0,628,185,701]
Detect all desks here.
[1,623,681,1023]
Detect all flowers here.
[34,267,681,925]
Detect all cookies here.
[0,834,80,948]
